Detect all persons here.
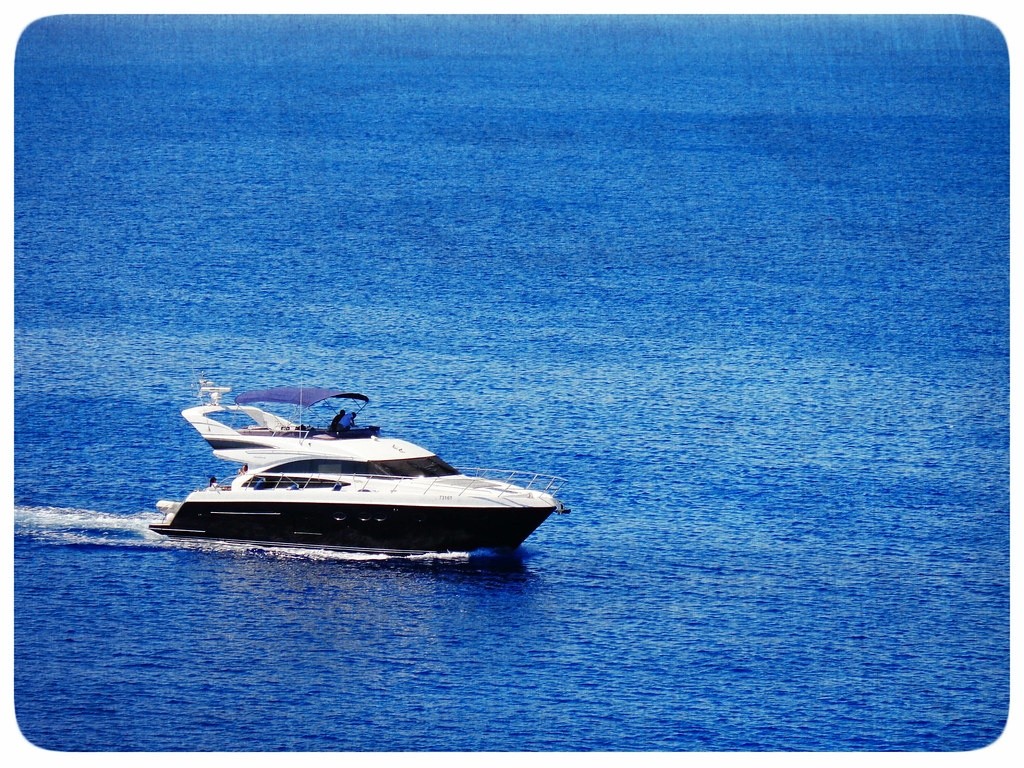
[209,475,221,490]
[328,409,358,433]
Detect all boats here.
[146,379,573,554]
